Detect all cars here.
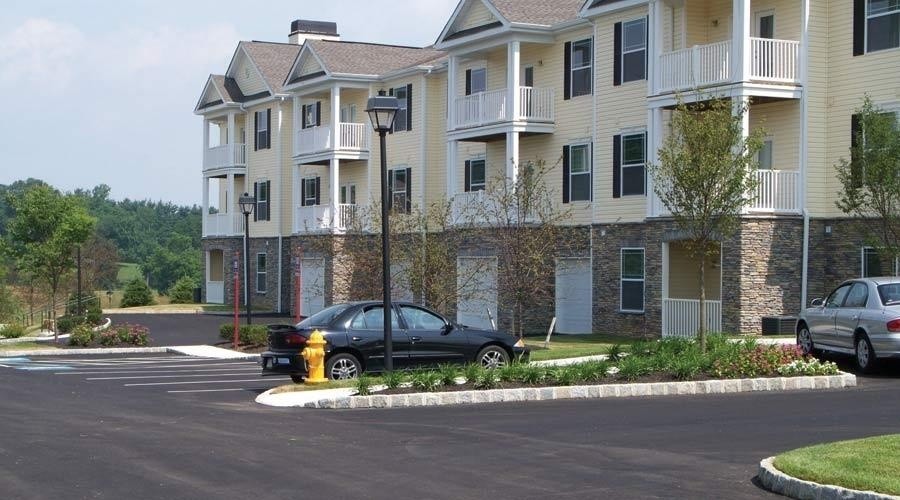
[794,275,900,370]
[262,300,532,383]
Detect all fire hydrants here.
[301,327,329,384]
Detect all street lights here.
[237,192,257,325]
[363,87,403,371]
